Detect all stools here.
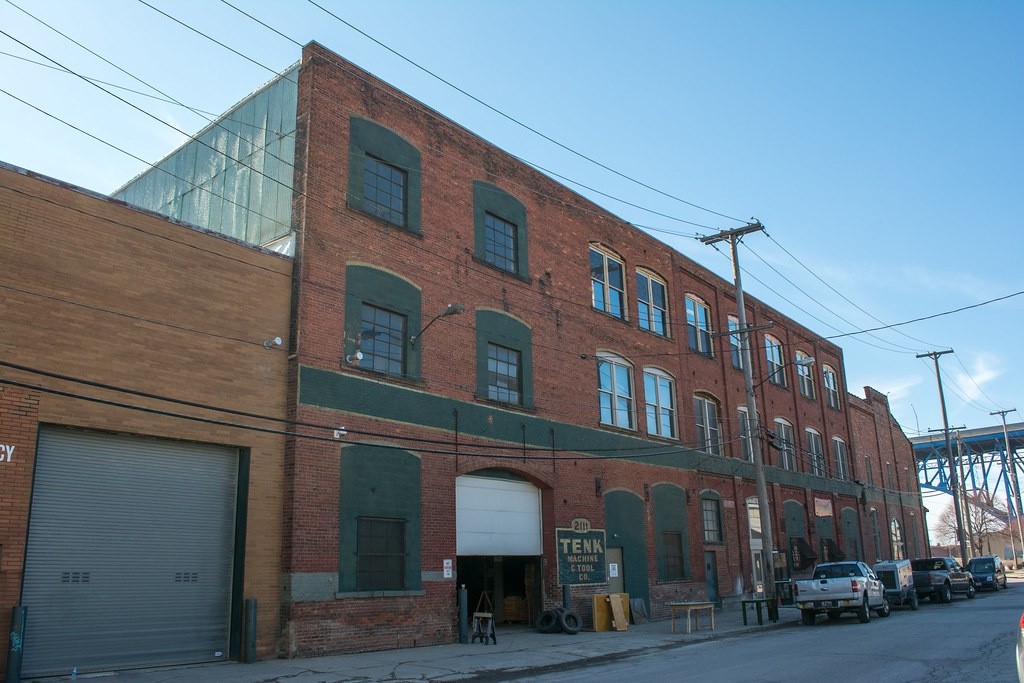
[471,612,497,645]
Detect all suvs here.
[966,555,1006,592]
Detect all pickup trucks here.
[910,557,975,605]
[794,561,890,624]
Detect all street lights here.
[411,305,466,348]
[753,355,816,393]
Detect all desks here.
[670,601,716,634]
[741,597,777,626]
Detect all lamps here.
[409,302,464,346]
[751,356,816,393]
